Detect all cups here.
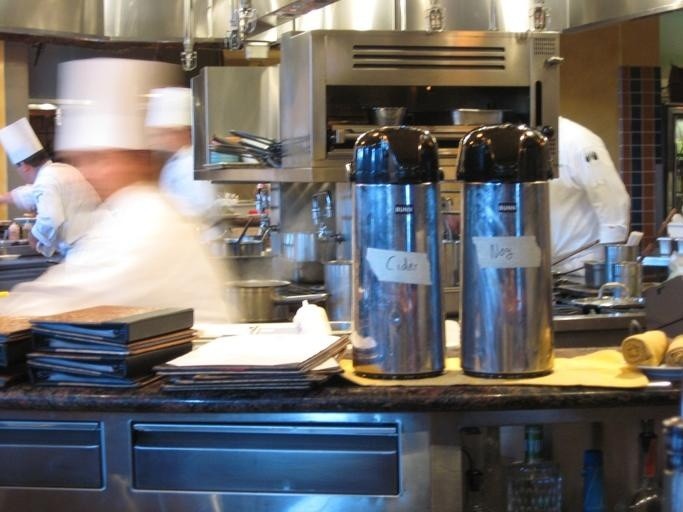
[583,244,643,299]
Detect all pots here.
[221,228,270,257]
[222,276,331,322]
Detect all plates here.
[624,362,682,379]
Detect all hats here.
[1,118,43,164]
[145,87,193,130]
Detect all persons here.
[146,127,227,258]
[0,148,104,262]
[0,148,245,326]
[547,113,633,286]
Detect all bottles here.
[348,124,446,381]
[8,218,33,242]
[501,420,566,512]
[456,122,558,378]
